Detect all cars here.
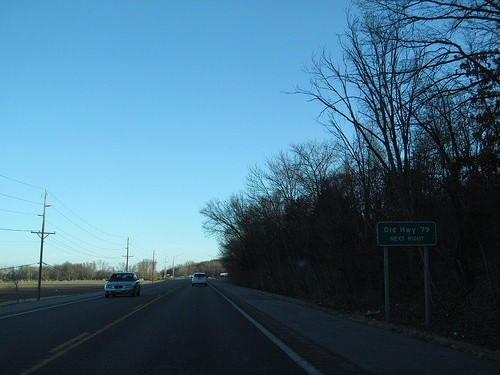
[192,271,208,286]
[104,270,139,297]
